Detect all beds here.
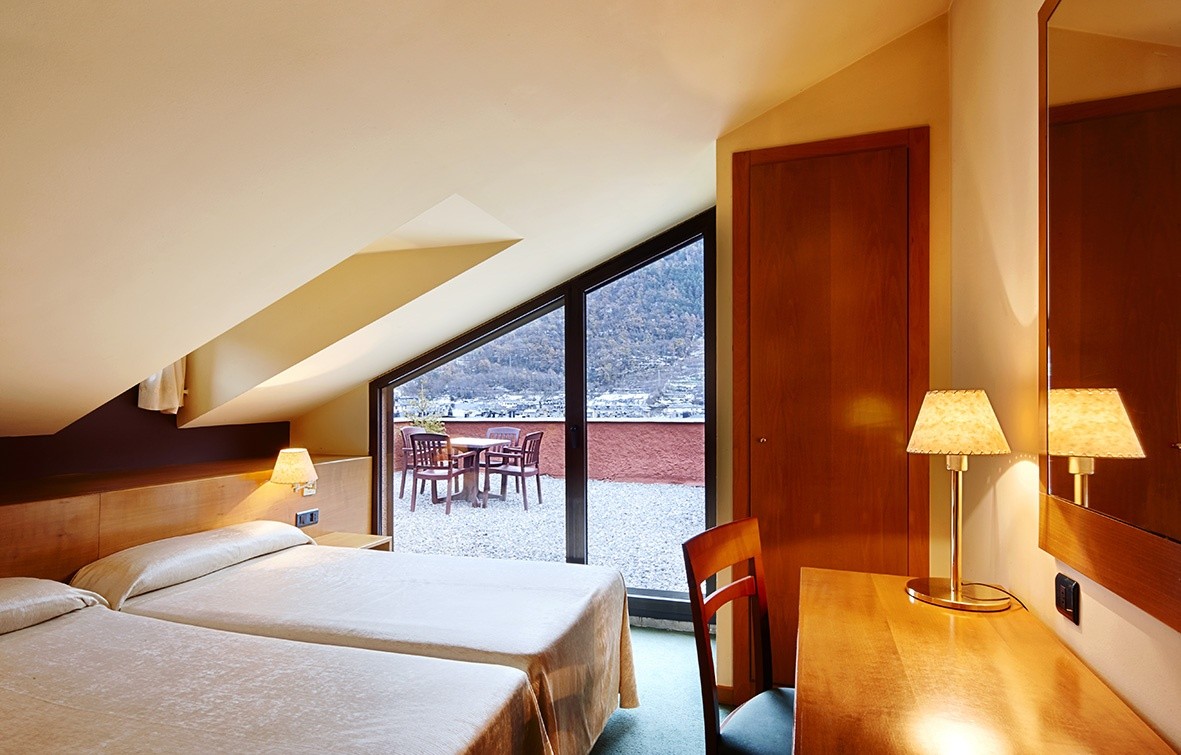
[0,520,640,755]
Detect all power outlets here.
[1056,572,1080,626]
[295,508,319,529]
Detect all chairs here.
[680,515,796,755]
[399,426,544,515]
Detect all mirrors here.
[1038,0,1181,635]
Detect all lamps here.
[905,390,1011,613]
[270,447,319,497]
[1048,387,1145,509]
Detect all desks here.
[792,566,1180,755]
[418,437,511,508]
